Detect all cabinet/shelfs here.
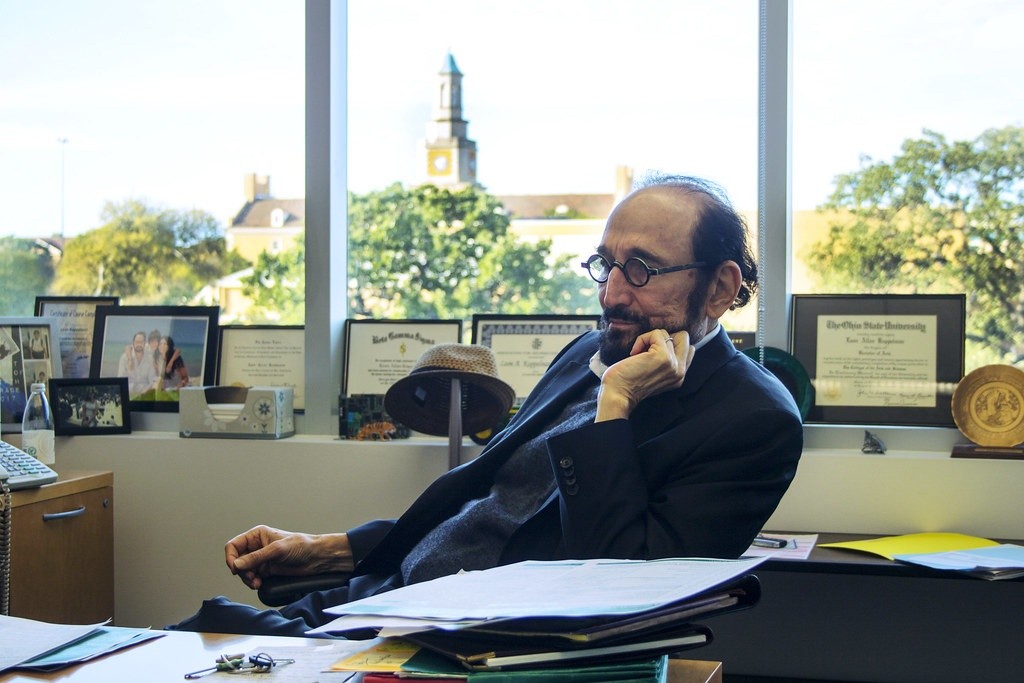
[1,459,118,627]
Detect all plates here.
[738,346,812,425]
[951,364,1024,448]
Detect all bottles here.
[21,382,56,471]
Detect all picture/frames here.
[0,317,63,435]
[89,305,220,413]
[48,375,132,435]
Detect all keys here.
[183,652,294,678]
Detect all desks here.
[0,613,722,683]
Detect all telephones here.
[0,440,59,490]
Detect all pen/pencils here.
[752,537,788,548]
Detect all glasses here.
[581,254,708,287]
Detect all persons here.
[26,330,46,358]
[161,174,808,641]
[117,331,189,398]
[35,372,48,384]
[81,390,120,429]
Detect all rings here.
[664,337,674,343]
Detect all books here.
[320,572,763,683]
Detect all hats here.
[383,343,517,436]
